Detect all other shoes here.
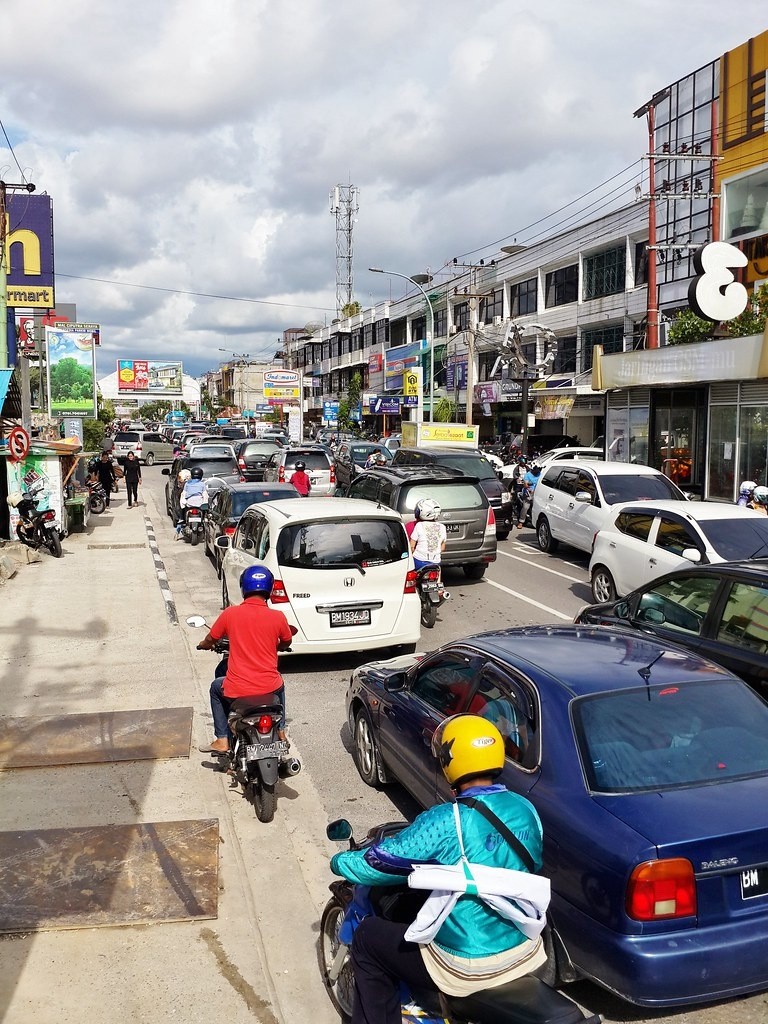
[517,523,522,528]
[173,531,178,541]
[133,502,138,507]
[127,506,133,509]
[177,519,184,523]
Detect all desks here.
[64,497,90,532]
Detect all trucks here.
[401,421,480,450]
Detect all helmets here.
[530,459,546,469]
[295,460,306,471]
[6,491,24,507]
[511,445,528,464]
[191,467,204,479]
[739,481,758,495]
[431,713,504,796]
[753,486,768,504]
[177,469,191,483]
[376,454,388,466]
[240,565,274,599]
[414,498,442,521]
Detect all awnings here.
[528,384,607,397]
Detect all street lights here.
[368,268,434,421]
[183,384,202,420]
[218,348,250,423]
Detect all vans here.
[335,463,497,579]
[390,448,513,539]
[213,498,421,657]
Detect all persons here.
[513,457,542,529]
[177,467,209,524]
[364,449,387,468]
[94,451,115,508]
[290,461,310,497]
[330,713,547,1024]
[105,422,126,438]
[737,480,768,515]
[405,498,446,570]
[99,432,115,453]
[123,451,142,509]
[197,564,292,752]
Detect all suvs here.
[510,435,582,459]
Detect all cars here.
[590,435,604,448]
[532,460,692,557]
[575,563,767,706]
[106,410,403,527]
[497,445,605,493]
[585,499,768,603]
[343,623,768,1009]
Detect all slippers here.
[199,741,220,752]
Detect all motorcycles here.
[181,507,205,545]
[88,481,110,515]
[9,488,69,557]
[317,818,603,1024]
[187,615,303,824]
[417,564,450,628]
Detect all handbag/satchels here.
[536,925,585,994]
